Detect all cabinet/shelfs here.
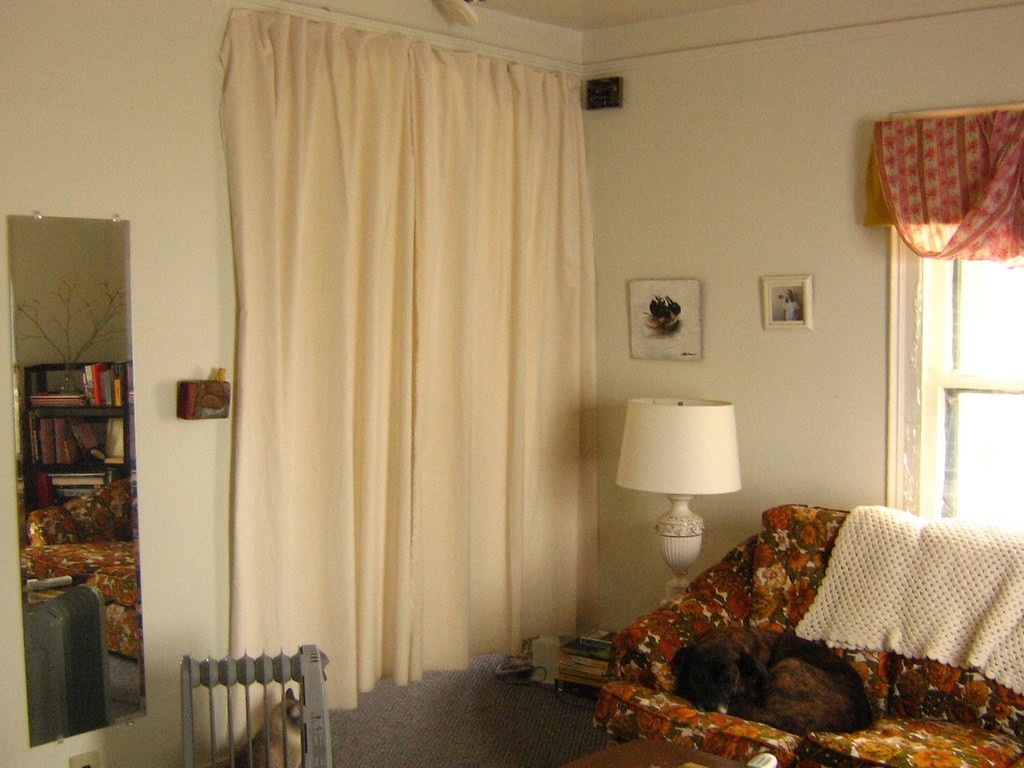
[23,360,128,518]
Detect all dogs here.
[234,687,301,768]
[669,627,872,734]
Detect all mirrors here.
[7,212,149,752]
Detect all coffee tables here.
[557,740,759,768]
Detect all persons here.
[781,289,802,321]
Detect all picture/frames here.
[760,275,815,332]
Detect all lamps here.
[446,0,482,27]
[614,396,745,607]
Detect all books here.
[51,470,107,502]
[557,623,620,686]
[28,408,98,463]
[106,414,125,463]
[82,362,126,408]
[28,393,85,406]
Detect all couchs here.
[20,480,138,661]
[593,503,1024,768]
